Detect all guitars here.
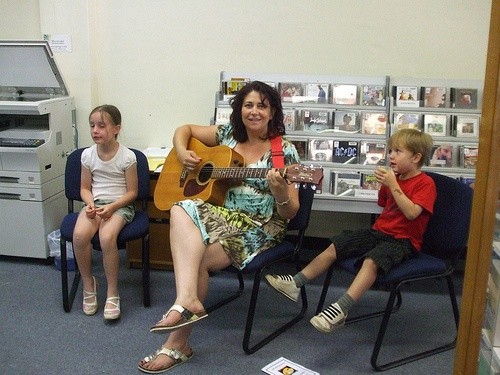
[150,137,326,211]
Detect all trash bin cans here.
[47,229,76,272]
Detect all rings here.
[267,178,270,181]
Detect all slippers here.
[150,304,208,334]
[138,345,194,373]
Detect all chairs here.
[60,146,149,313]
[316,172,473,372]
[205,164,315,355]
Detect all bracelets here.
[275,195,291,205]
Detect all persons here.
[73,104,138,320]
[138,81,300,373]
[264,129,437,333]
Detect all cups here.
[377,165,389,183]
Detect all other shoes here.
[104,294,120,319]
[82,276,98,315]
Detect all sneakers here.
[309,303,348,334]
[264,274,301,303]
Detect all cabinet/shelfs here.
[126,157,173,272]
[210,71,484,214]
